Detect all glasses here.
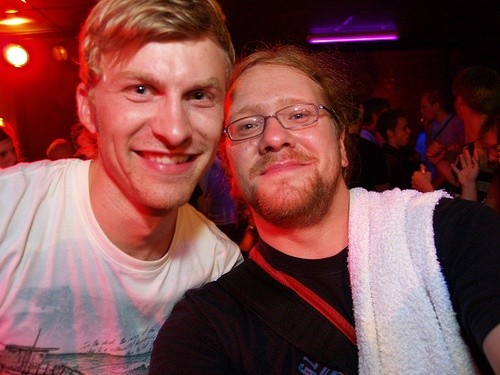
[221,102,341,142]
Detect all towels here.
[347,187,474,375]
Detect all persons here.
[0,0,244,375]
[149,43,500,375]
[341,69,500,212]
[0,118,83,170]
